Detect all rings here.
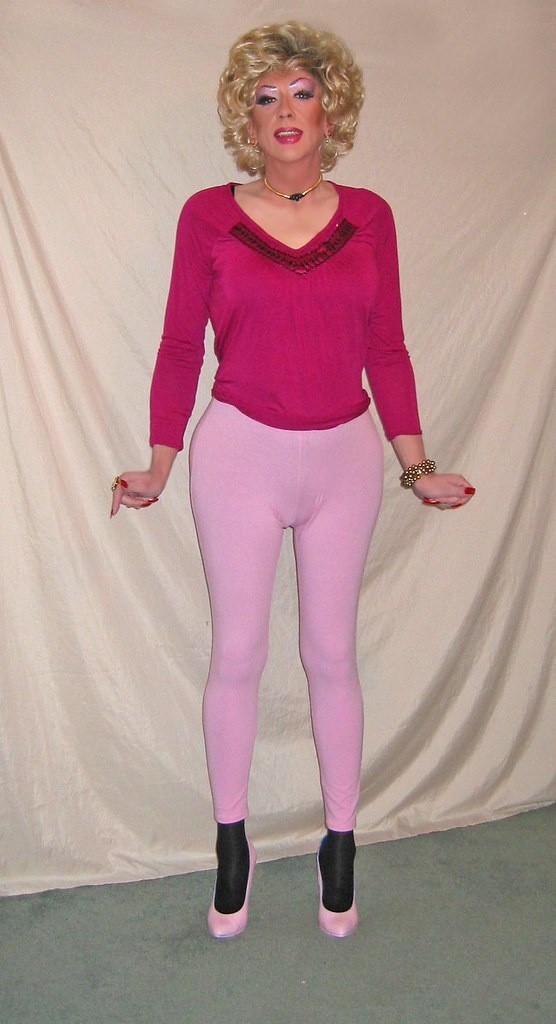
[111,476,120,491]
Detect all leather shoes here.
[316,848,358,937]
[208,838,257,938]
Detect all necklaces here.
[264,171,323,201]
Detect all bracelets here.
[401,459,437,488]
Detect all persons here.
[110,24,476,937]
